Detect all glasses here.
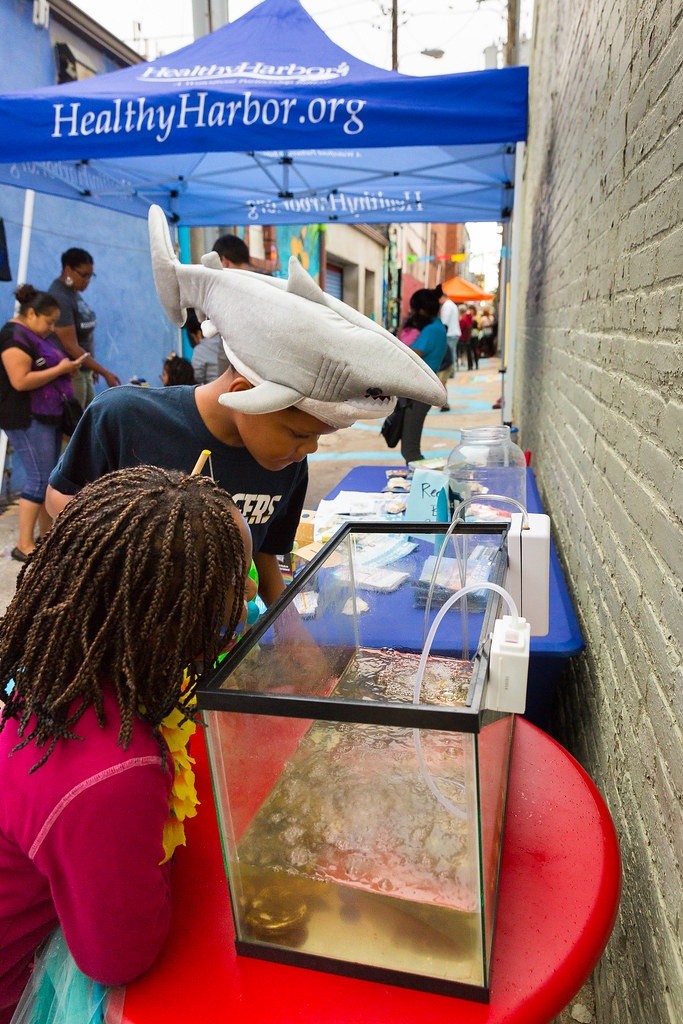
[72,268,96,280]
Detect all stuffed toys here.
[147,204,448,427]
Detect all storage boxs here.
[275,522,348,592]
[194,520,518,1005]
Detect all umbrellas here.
[441,276,494,302]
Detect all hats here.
[147,204,447,430]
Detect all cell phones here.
[76,352,90,361]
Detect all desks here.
[220,466,586,660]
[103,677,623,1024]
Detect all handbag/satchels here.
[381,397,407,448]
[60,399,83,438]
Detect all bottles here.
[447,425,526,523]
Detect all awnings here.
[0,0,528,455]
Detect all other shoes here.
[36,536,45,544]
[492,398,502,408]
[11,548,28,562]
[442,403,449,411]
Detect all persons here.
[0,463,252,1024]
[0,234,497,704]
